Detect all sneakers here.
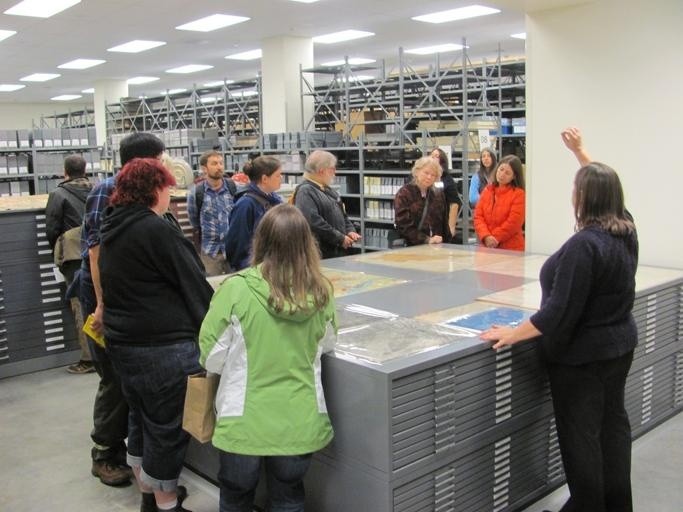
[91,459,132,485]
[67,360,97,374]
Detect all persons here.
[224,156,287,270]
[287,149,363,257]
[197,202,340,512]
[472,154,525,249]
[468,148,497,209]
[407,147,463,238]
[46,155,97,374]
[394,156,453,245]
[479,124,641,511]
[79,133,164,487]
[97,159,214,511]
[185,152,245,276]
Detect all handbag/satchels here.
[387,229,409,248]
[53,225,83,266]
[181,371,221,444]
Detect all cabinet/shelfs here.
[0,37,525,254]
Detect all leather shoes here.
[140,485,187,512]
[156,502,192,511]
